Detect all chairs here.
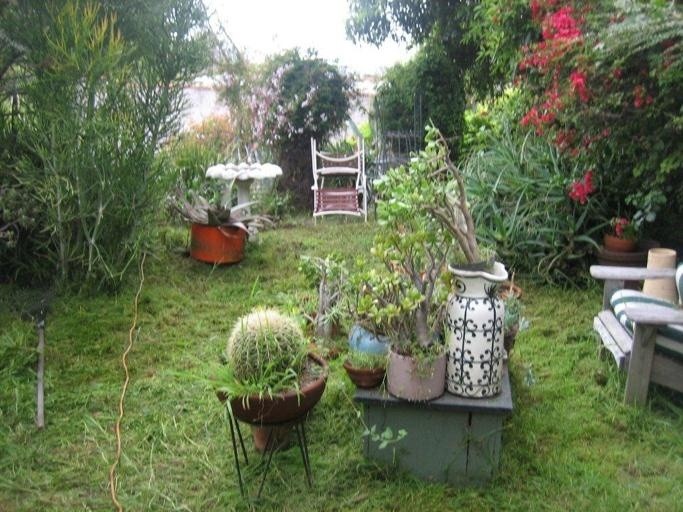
[591,264,683,412]
[309,137,368,224]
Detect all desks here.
[350,360,514,483]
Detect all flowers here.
[608,215,635,239]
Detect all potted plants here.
[169,305,329,423]
[165,170,276,263]
[416,129,507,396]
[347,279,399,356]
[342,351,386,388]
[346,159,447,401]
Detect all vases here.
[499,285,520,350]
[251,424,294,452]
[604,234,637,252]
